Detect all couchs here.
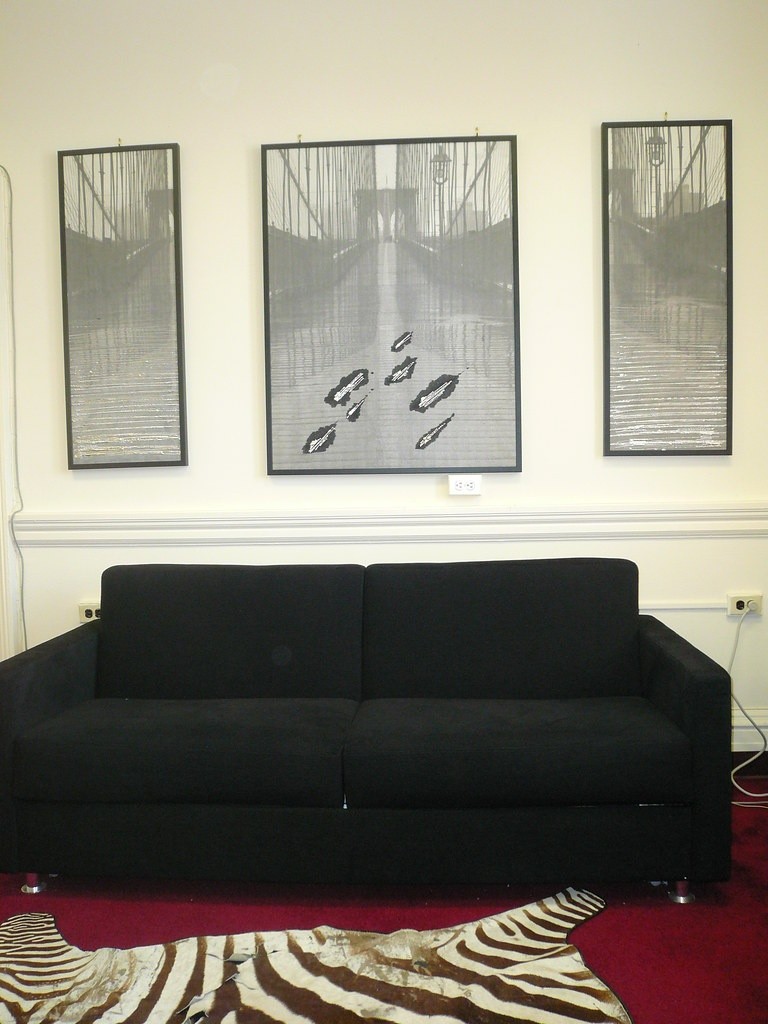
[0,557,733,903]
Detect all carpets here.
[0,887,633,1024]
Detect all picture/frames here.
[601,119,733,456]
[58,143,191,467]
[262,135,524,477]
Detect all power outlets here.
[447,475,483,495]
[728,593,762,616]
[79,602,101,623]
